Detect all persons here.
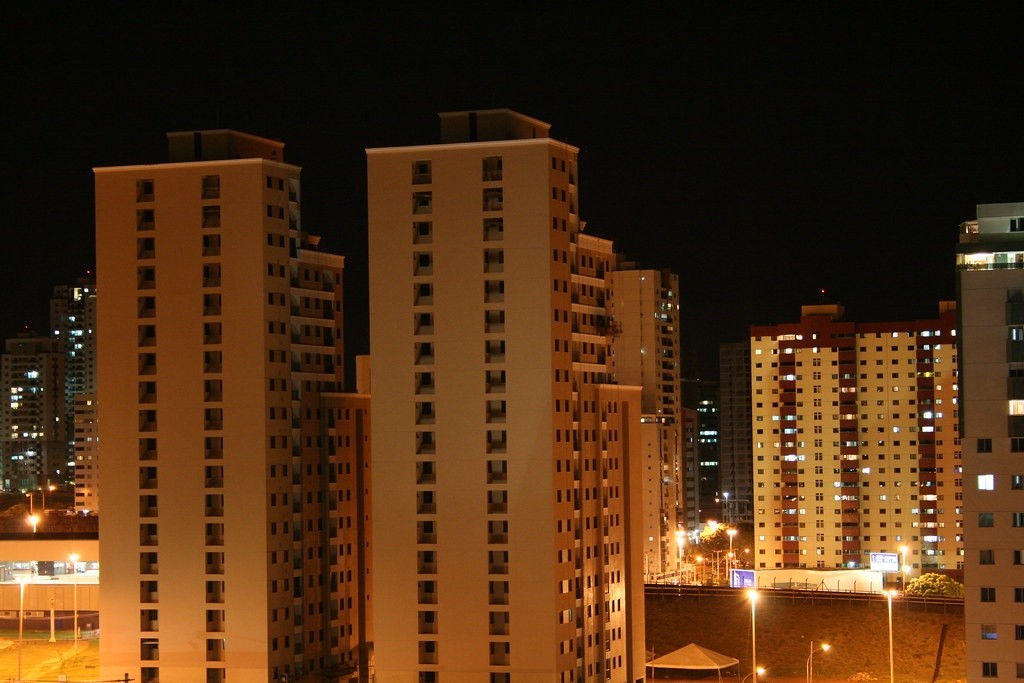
[31,566,36,575]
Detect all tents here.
[647,642,741,683]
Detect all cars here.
[715,559,733,567]
[673,577,689,585]
[66,564,77,574]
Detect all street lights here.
[71,554,80,655]
[806,644,828,683]
[900,546,908,595]
[728,530,735,569]
[11,569,32,680]
[747,590,758,683]
[676,532,684,584]
[726,553,732,581]
[28,491,33,513]
[883,589,896,683]
